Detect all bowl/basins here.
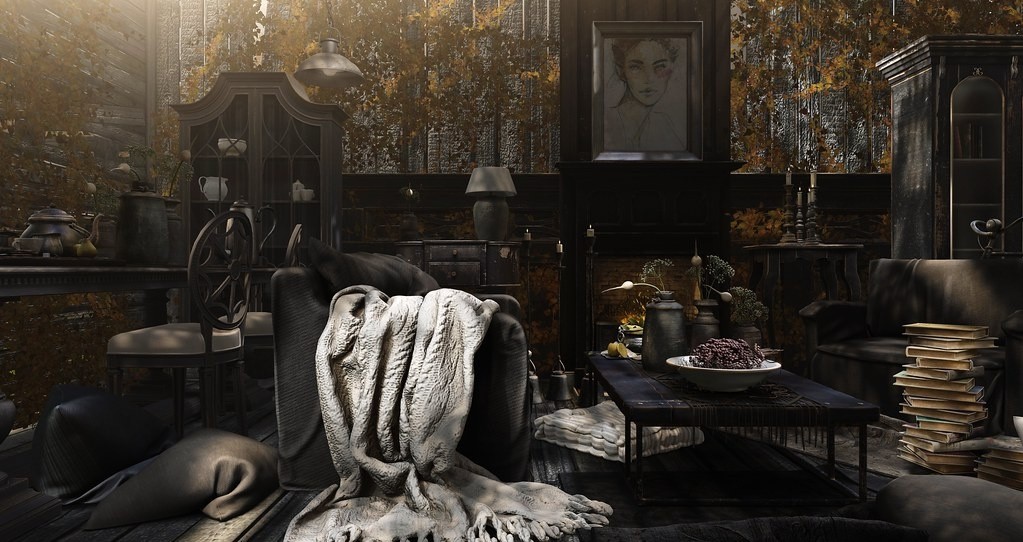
[666,356,782,393]
[218,139,247,156]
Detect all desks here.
[588,352,880,507]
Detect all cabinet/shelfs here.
[952,112,1002,256]
[191,153,320,318]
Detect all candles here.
[786,166,817,206]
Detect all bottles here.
[77,237,97,258]
[31,233,63,257]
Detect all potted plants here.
[728,287,768,350]
[686,254,735,350]
[155,154,195,266]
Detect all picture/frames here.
[590,20,703,162]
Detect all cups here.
[11,238,44,255]
[301,188,314,200]
[289,190,301,200]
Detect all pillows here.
[305,237,440,301]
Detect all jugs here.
[199,176,228,201]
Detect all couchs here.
[796,257,1023,424]
[272,267,534,491]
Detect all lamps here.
[465,166,517,240]
[292,0,365,86]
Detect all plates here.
[601,348,637,359]
[0,246,16,255]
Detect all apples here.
[608,341,620,356]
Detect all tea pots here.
[205,195,279,264]
[0,203,106,255]
[293,179,305,194]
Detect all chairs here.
[104,211,255,443]
[218,222,309,416]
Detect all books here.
[955,121,984,159]
[893,323,1023,493]
